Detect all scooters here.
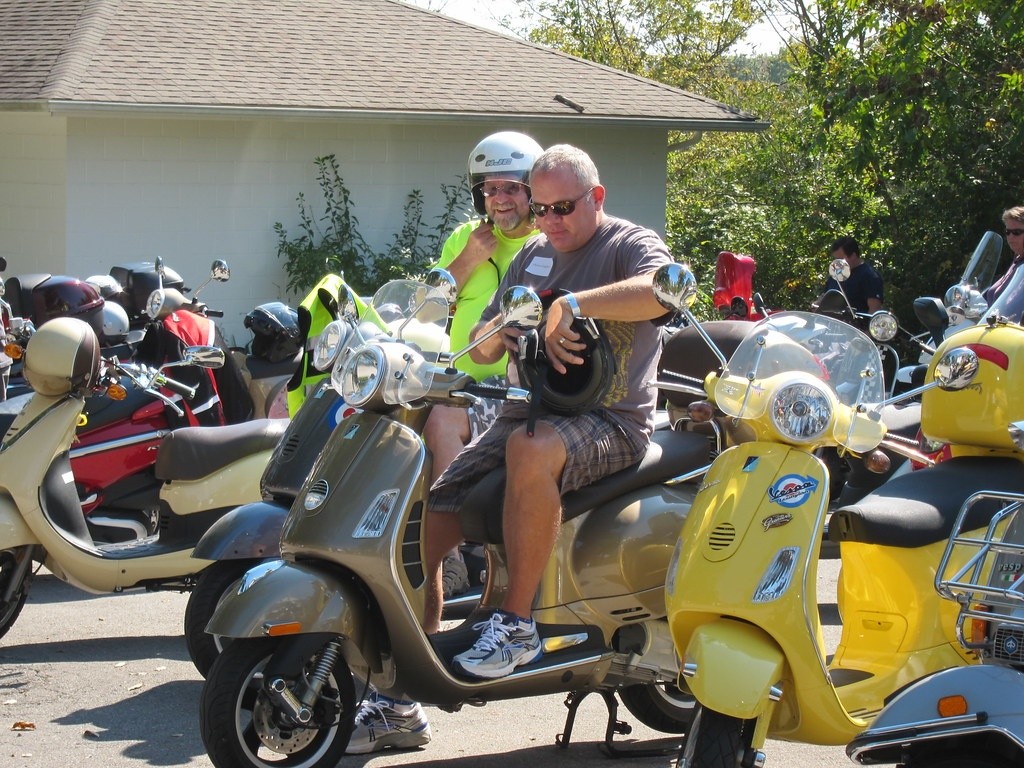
[1,205,1019,768]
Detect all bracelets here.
[563,292,581,318]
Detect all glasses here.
[480,182,525,197]
[528,186,594,217]
[1006,228,1024,236]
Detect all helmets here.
[511,286,620,417]
[466,131,545,214]
[245,302,304,364]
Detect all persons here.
[807,234,885,341]
[339,140,678,757]
[414,129,545,601]
[980,205,1024,327]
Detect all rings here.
[558,337,566,345]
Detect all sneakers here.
[344,690,432,753]
[442,552,471,599]
[450,608,543,679]
